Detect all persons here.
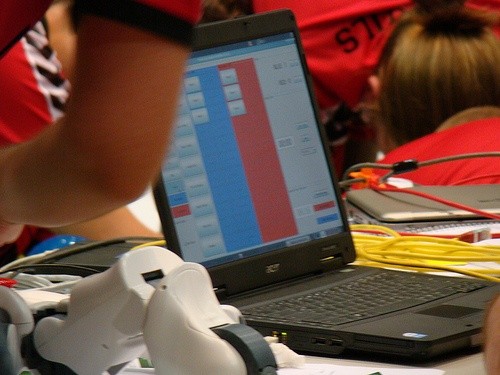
[1,1,201,245]
[340,8,499,197]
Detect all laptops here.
[346,183,500,223]
[22,240,168,281]
[152,9,500,361]
